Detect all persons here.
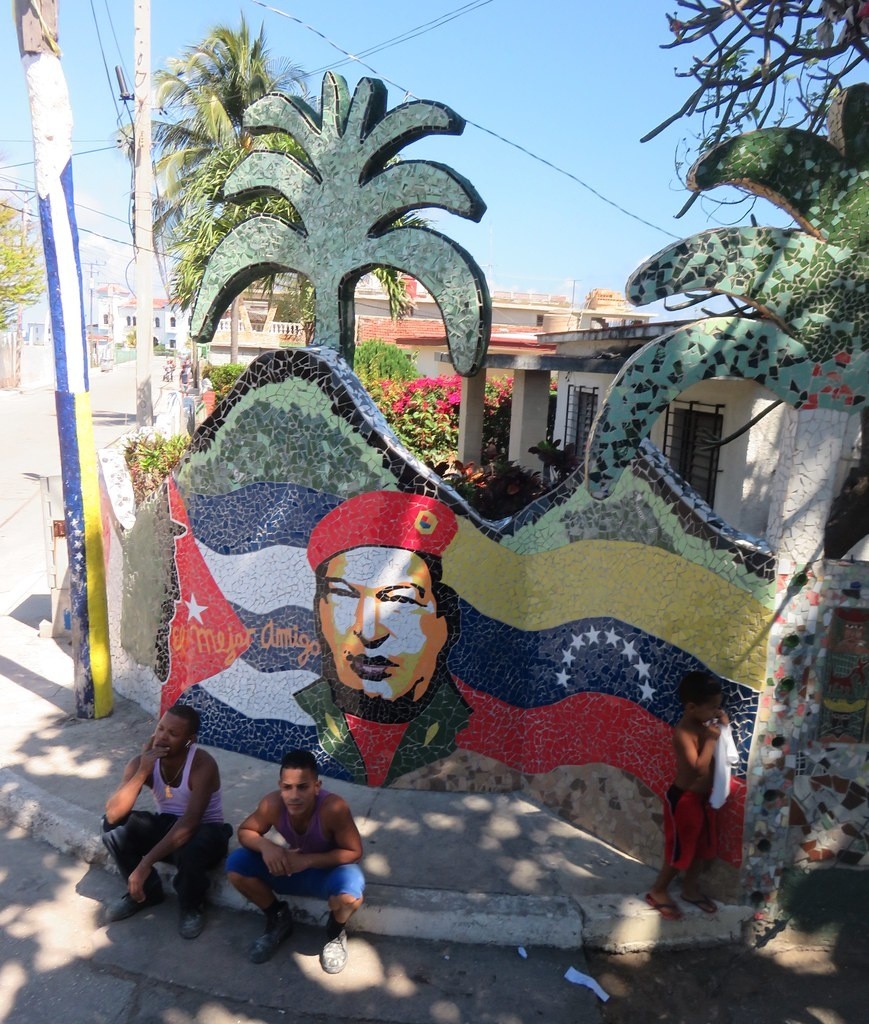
[226,750,364,974]
[201,373,213,394]
[101,705,233,939]
[646,672,728,919]
[179,365,190,398]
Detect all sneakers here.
[178,900,208,939]
[248,901,291,961]
[321,924,349,973]
[104,880,165,920]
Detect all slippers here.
[646,892,682,920]
[680,893,717,913]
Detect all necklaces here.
[161,759,186,798]
